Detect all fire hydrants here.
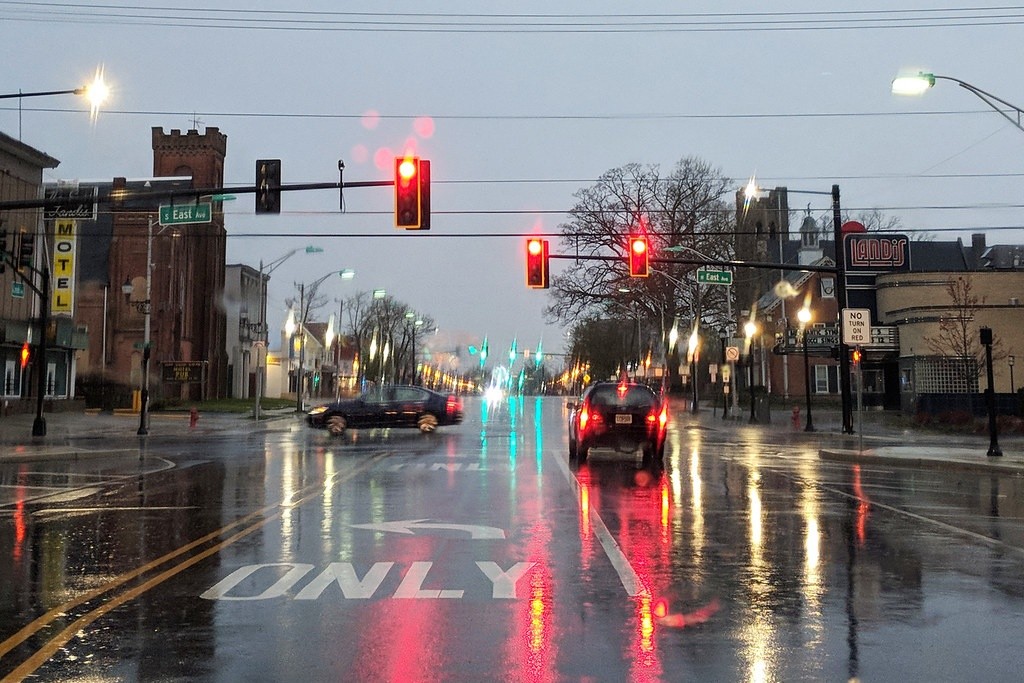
[791,405,800,430]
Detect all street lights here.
[688,333,699,414]
[335,289,386,402]
[745,323,760,424]
[122,195,237,434]
[297,268,356,413]
[663,246,741,422]
[648,265,700,409]
[618,289,665,402]
[797,308,815,432]
[745,182,854,434]
[254,245,323,421]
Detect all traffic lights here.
[536,349,543,363]
[630,238,649,277]
[526,239,544,288]
[394,157,421,229]
[480,345,488,359]
[18,228,35,272]
[853,349,867,362]
[0,223,6,273]
[20,343,34,366]
[468,345,476,355]
[509,348,517,359]
[190,407,201,429]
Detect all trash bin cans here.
[754,392,770,423]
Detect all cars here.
[304,384,465,437]
[567,381,668,464]
